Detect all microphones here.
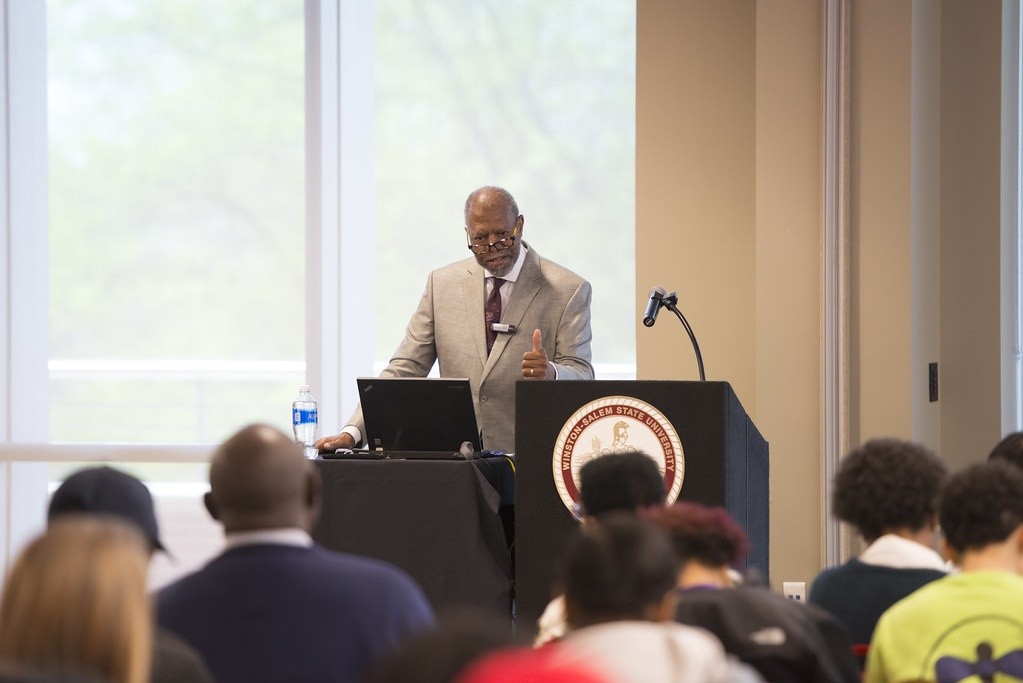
[643,287,666,327]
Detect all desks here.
[311,457,515,625]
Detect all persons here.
[308,187,593,459]
[0,419,1022,683]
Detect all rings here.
[530,369,534,375]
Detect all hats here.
[48,466,168,557]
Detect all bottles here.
[292,385,318,459]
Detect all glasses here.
[466,224,517,254]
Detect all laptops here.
[356,377,506,460]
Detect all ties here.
[484,278,507,356]
